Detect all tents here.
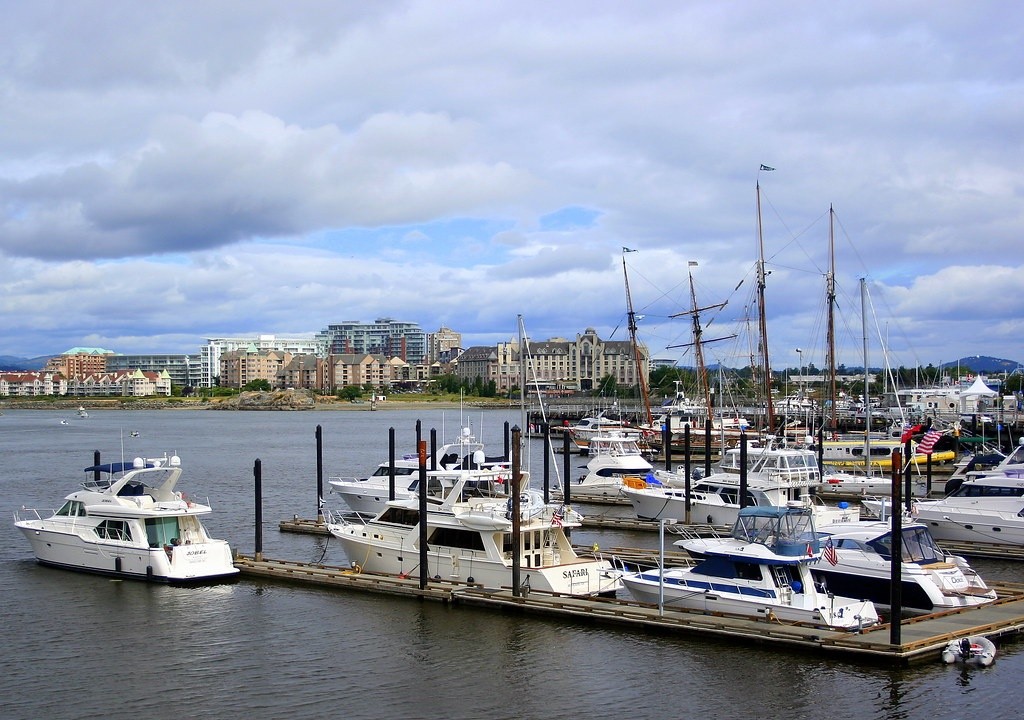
[961,374,997,413]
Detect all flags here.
[623,247,638,252]
[553,506,565,530]
[917,430,943,456]
[642,430,660,436]
[760,164,775,171]
[806,542,814,558]
[824,537,838,566]
[688,261,698,266]
[900,425,920,443]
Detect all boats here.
[623,542,879,629]
[541,456,651,504]
[128,430,141,439]
[76,405,90,419]
[11,425,241,588]
[338,386,551,507]
[325,500,623,597]
[712,446,928,499]
[392,467,584,535]
[671,503,998,611]
[584,437,643,457]
[612,471,860,529]
[863,436,1024,559]
[60,419,69,425]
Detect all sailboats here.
[555,166,895,443]
